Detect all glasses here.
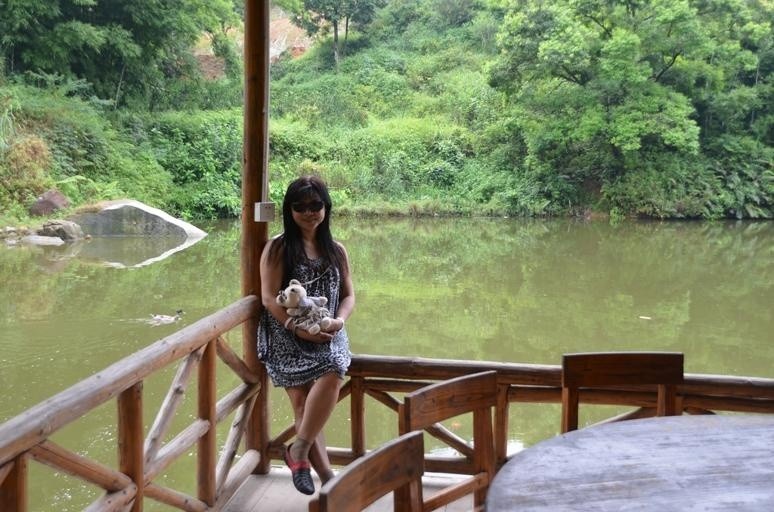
[290,200,324,213]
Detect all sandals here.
[283,442,314,495]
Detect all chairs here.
[404,369,500,511]
[309,429,423,511]
[561,353,685,432]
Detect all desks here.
[486,413,774,511]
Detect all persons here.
[257,177,355,495]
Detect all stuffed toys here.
[276,279,331,335]
